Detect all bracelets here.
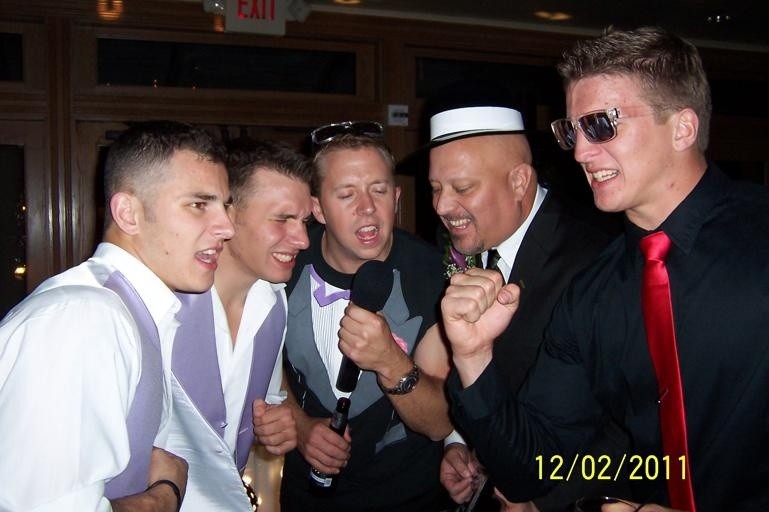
[631,502,647,512]
[149,479,182,511]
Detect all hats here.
[429,106,526,149]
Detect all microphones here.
[336,259,394,392]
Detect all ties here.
[640,229,698,512]
[485,249,506,285]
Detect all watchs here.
[377,359,420,396]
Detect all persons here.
[424,105,617,512]
[275,115,457,512]
[0,116,239,510]
[180,134,313,510]
[438,17,768,512]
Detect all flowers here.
[439,233,476,281]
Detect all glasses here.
[550,105,683,150]
[310,120,384,144]
